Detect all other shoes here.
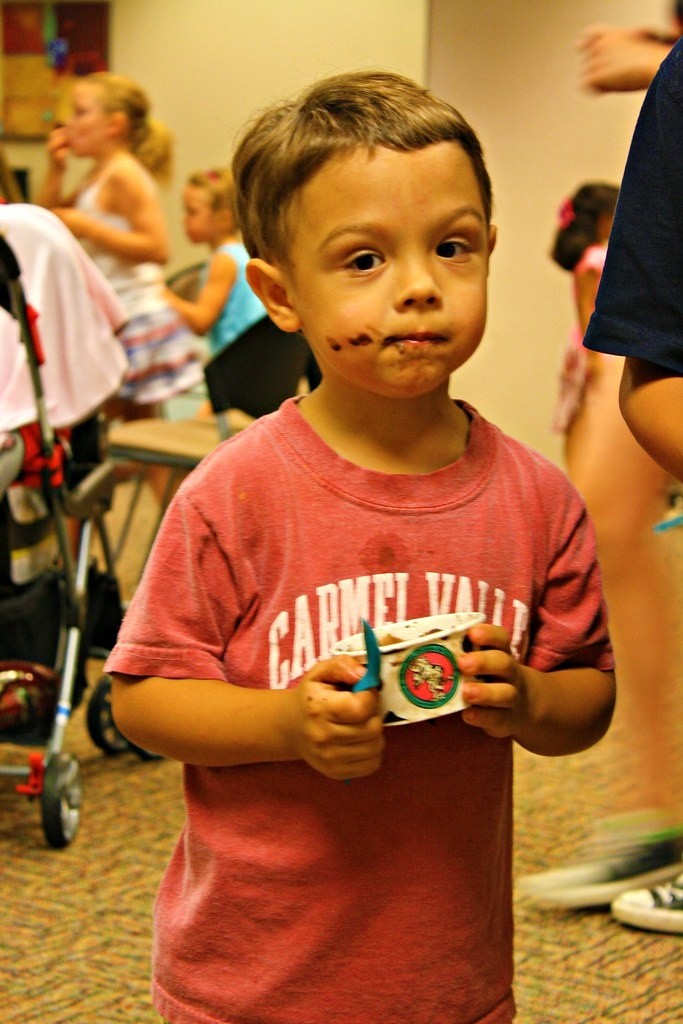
[612,873,683,933]
[521,834,683,911]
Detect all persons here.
[582,36,683,484]
[550,182,619,467]
[158,170,265,359]
[514,403,683,936]
[33,72,206,515]
[101,72,619,1023]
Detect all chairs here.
[110,309,323,585]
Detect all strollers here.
[0,198,166,851]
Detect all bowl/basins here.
[330,611,490,731]
